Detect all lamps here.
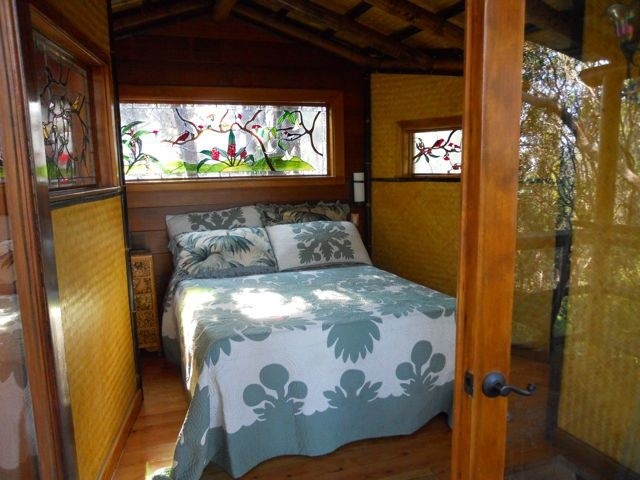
[354,172,366,212]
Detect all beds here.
[153,265,458,479]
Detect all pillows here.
[263,200,350,224]
[168,227,278,280]
[166,203,266,239]
[265,221,374,272]
